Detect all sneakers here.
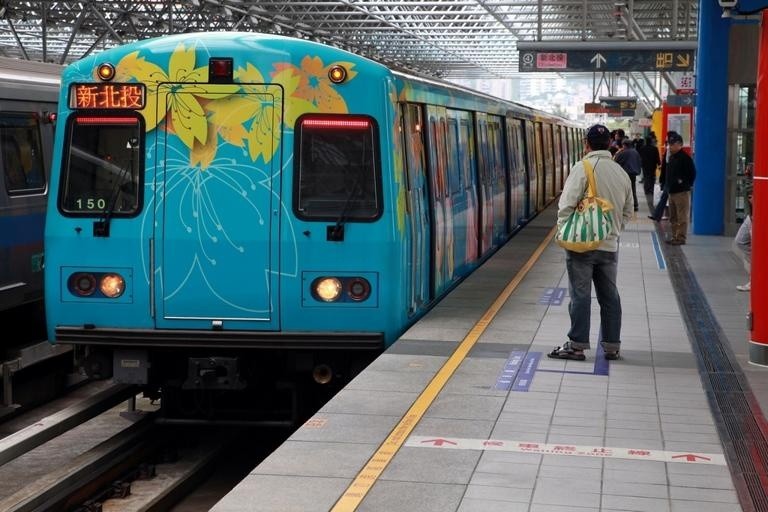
[736,282,750,291]
[665,240,685,245]
[648,216,660,222]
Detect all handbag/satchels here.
[555,198,614,253]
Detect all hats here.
[587,125,611,138]
[669,135,682,143]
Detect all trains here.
[43,31,586,422]
[1,56,87,417]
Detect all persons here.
[734,161,752,291]
[547,124,634,360]
[665,135,696,246]
[648,131,677,222]
[609,129,662,212]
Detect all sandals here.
[604,351,619,360]
[547,341,585,359]
